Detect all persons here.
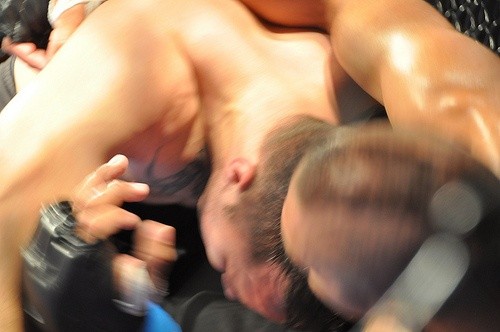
[1,0,391,332]
[17,0,498,332]
